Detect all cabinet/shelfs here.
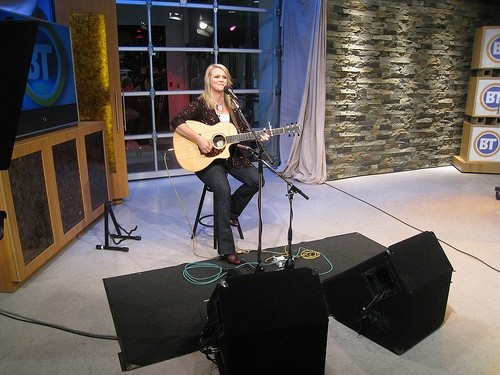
[0,121,111,293]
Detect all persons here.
[173,63,271,264]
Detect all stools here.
[191,174,244,240]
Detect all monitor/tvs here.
[0,8,79,141]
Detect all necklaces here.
[213,98,224,111]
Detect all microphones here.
[236,144,259,154]
[223,85,240,101]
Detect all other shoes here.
[229,219,238,226]
[224,254,240,264]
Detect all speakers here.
[0,16,38,169]
[206,266,328,375]
[322,231,453,355]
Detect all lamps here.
[196,21,214,37]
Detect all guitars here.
[172,120,303,173]
